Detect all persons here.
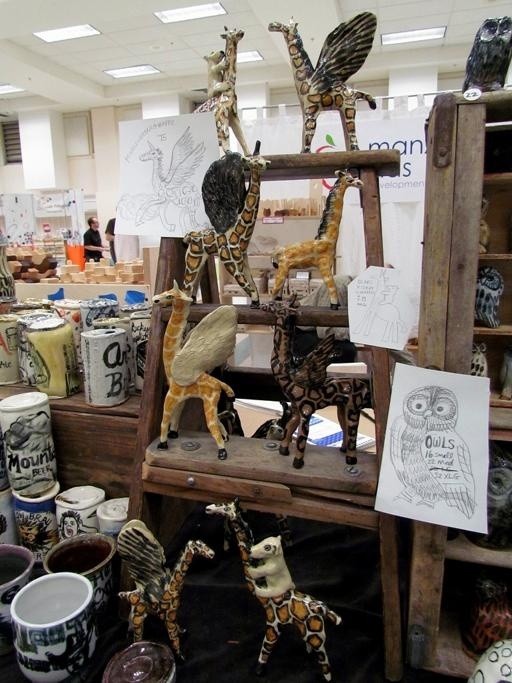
[104,217,116,265]
[81,214,110,263]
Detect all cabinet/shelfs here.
[408,90,511,664]
[3,240,148,308]
[0,358,211,603]
[116,148,411,664]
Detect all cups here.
[0,296,150,683]
